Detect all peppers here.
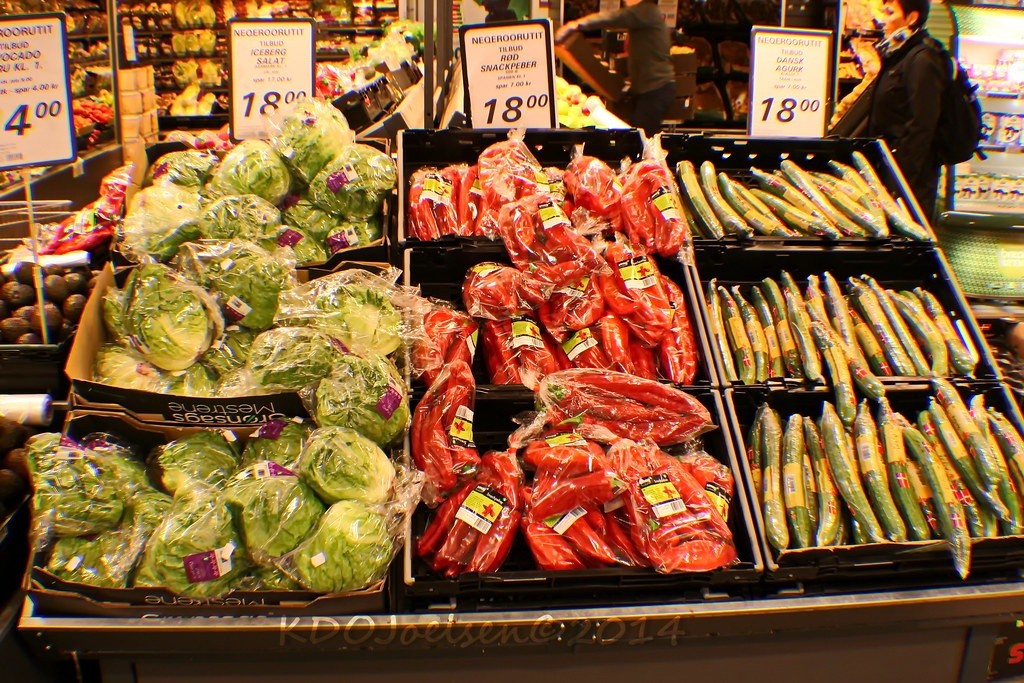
[314,62,358,99]
[405,141,738,574]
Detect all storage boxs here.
[403,248,720,390]
[652,128,942,251]
[402,390,761,601]
[726,383,1024,574]
[61,262,395,422]
[109,139,392,268]
[396,128,688,249]
[19,413,392,619]
[685,243,1003,391]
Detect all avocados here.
[0,260,94,346]
[0,416,37,518]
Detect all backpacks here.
[901,44,989,165]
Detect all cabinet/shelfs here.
[0,0,405,198]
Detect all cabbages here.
[170,4,219,89]
[22,99,414,599]
[350,19,426,70]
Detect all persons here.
[555,0,676,139]
[860,0,951,223]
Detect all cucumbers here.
[671,149,1024,581]
